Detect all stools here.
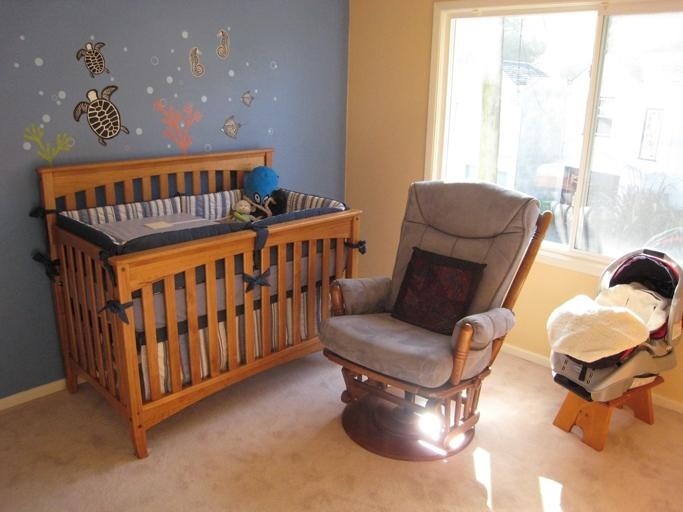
[553,377,663,451]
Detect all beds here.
[35,147,364,459]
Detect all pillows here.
[391,245,487,335]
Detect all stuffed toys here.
[243,190,288,219]
[243,165,280,208]
[233,200,257,224]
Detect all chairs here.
[319,179,553,462]
